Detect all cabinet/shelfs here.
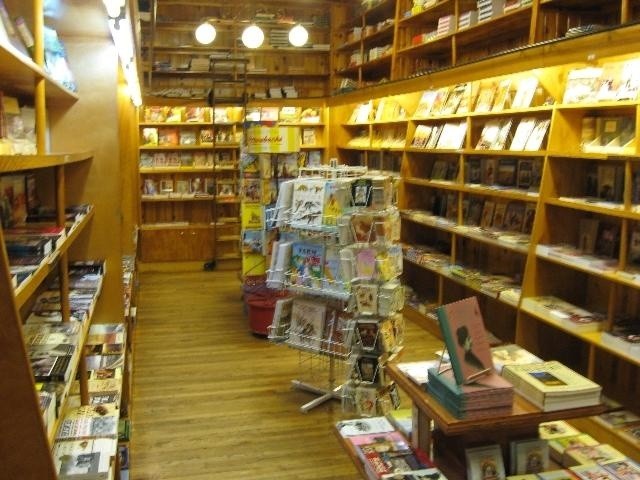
[328,2,639,479]
[141,2,326,262]
[1,1,142,478]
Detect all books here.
[241,1,640,479]
[0,5,136,479]
[139,56,267,197]
